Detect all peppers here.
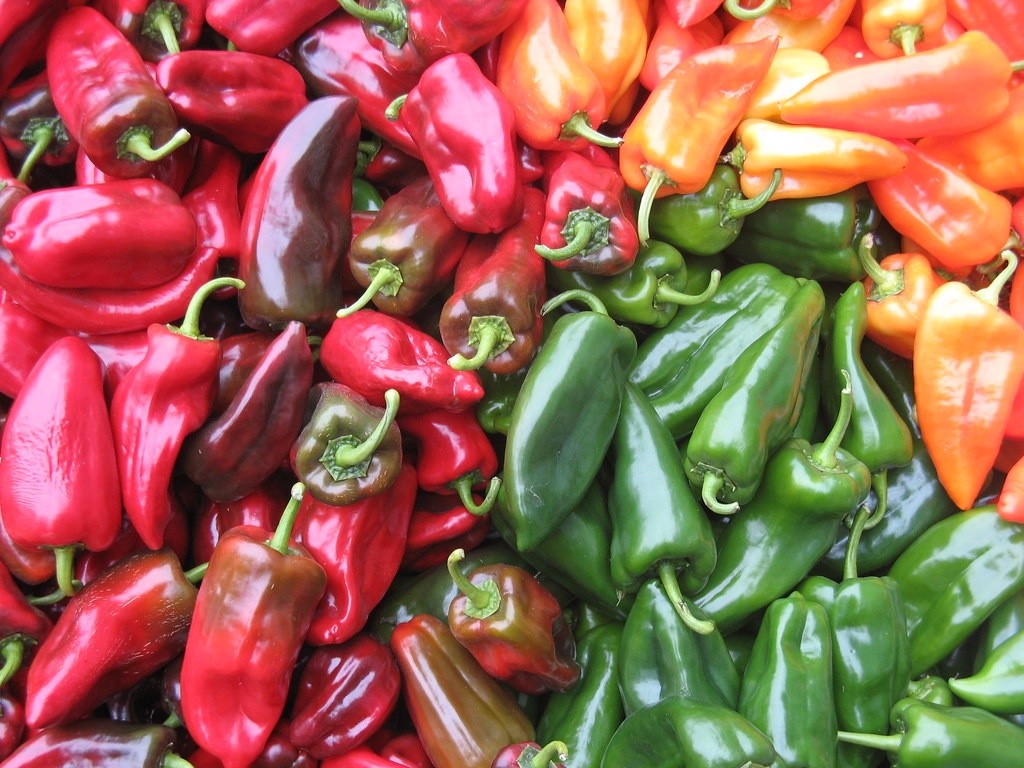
[0,0,1024,768]
